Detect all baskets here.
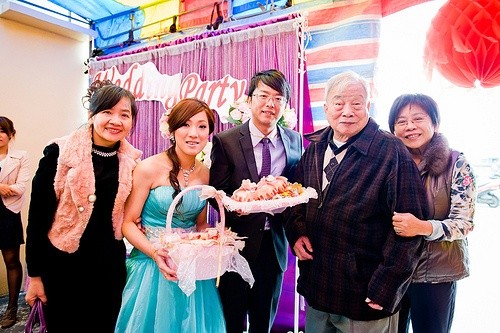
[163,183,236,280]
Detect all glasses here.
[249,94,288,104]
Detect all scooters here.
[477,178,500,208]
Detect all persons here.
[208,69,303,333]
[24,80,142,333]
[0,115,30,325]
[114,98,227,333]
[286,70,429,332]
[388,93,477,333]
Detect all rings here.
[395,229,401,236]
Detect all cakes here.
[231,175,304,202]
[180,226,238,247]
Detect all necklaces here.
[91,144,119,160]
[167,147,199,186]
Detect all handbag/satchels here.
[23,300,47,333]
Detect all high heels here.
[2,305,18,328]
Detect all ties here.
[259,138,271,177]
